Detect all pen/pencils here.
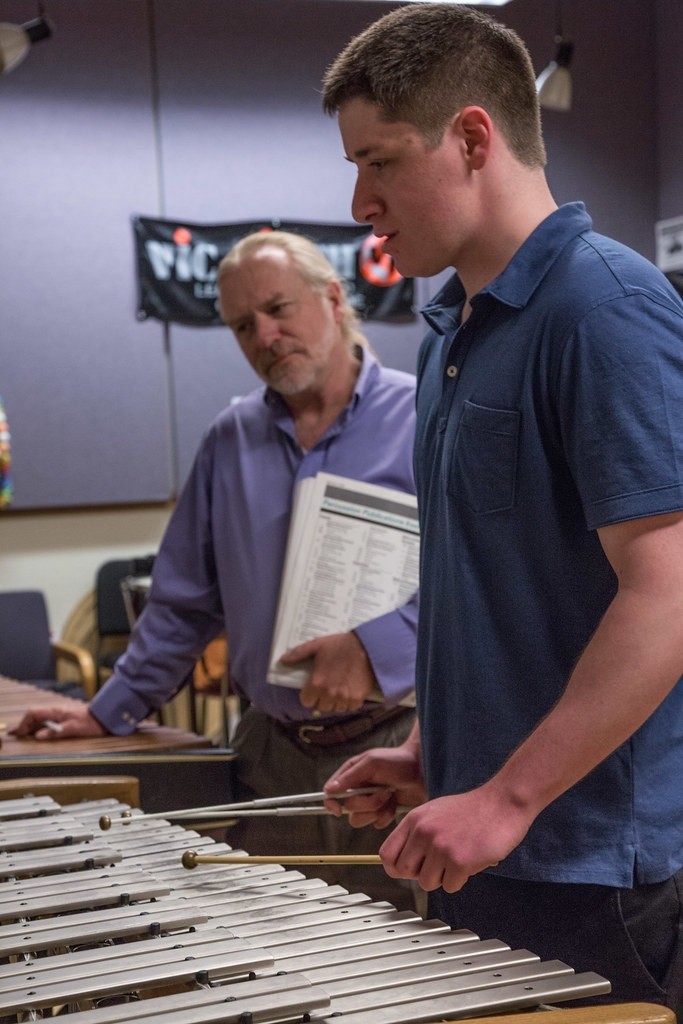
[42,719,64,734]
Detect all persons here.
[318,0,683,1024]
[11,229,421,919]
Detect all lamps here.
[532,1,577,111]
[0,0,53,73]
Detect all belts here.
[241,705,415,749]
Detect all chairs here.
[96,555,164,726]
[0,590,97,701]
